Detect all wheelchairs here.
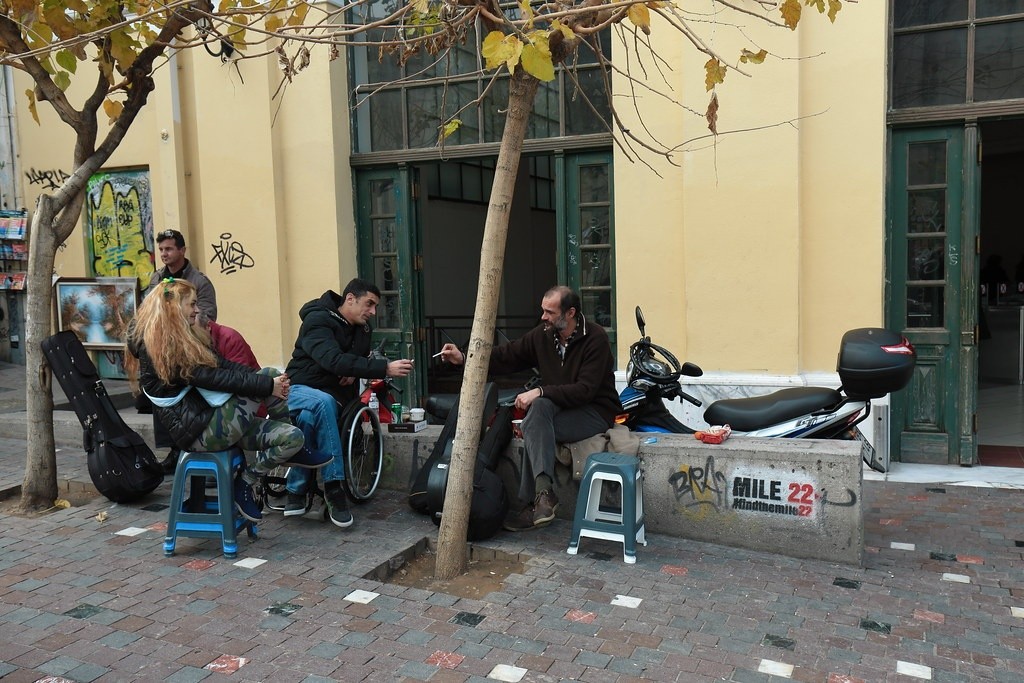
[255,376,404,511]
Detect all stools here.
[567,452,647,563]
[162,444,258,560]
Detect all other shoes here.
[204,476,218,487]
[160,449,181,475]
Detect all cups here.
[512,419,524,440]
[401,406,410,423]
[410,408,425,423]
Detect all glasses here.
[164,231,178,241]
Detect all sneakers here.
[282,443,335,467]
[323,486,353,527]
[284,490,306,516]
[532,487,559,525]
[502,502,554,531]
[232,477,263,522]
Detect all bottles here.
[368,393,380,428]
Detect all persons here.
[122,275,334,523]
[285,278,414,527]
[441,283,625,531]
[133,229,218,414]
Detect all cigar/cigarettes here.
[410,358,415,364]
[432,352,442,358]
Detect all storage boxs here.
[836,327,918,402]
[388,421,428,433]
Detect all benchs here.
[314,421,866,567]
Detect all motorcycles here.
[615,305,917,473]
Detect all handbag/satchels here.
[196,385,235,407]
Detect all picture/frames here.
[51,276,140,350]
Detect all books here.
[0,241,28,259]
[0,271,26,290]
[0,217,27,239]
